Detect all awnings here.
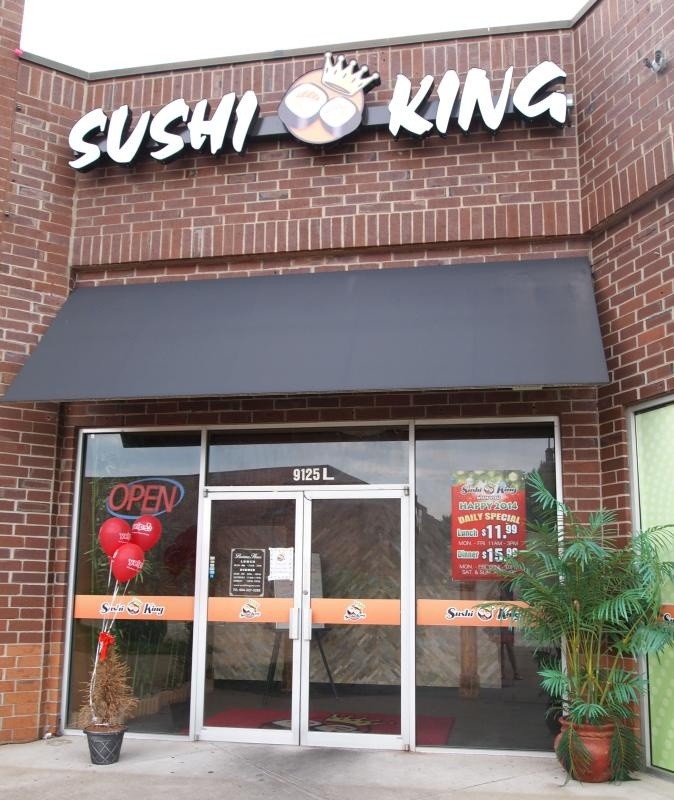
[0,255,613,405]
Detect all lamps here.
[640,50,665,73]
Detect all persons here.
[500,581,524,684]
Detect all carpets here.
[178,706,455,748]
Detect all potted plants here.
[472,468,674,788]
[78,648,140,765]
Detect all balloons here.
[98,517,132,559]
[109,544,145,584]
[131,515,163,552]
